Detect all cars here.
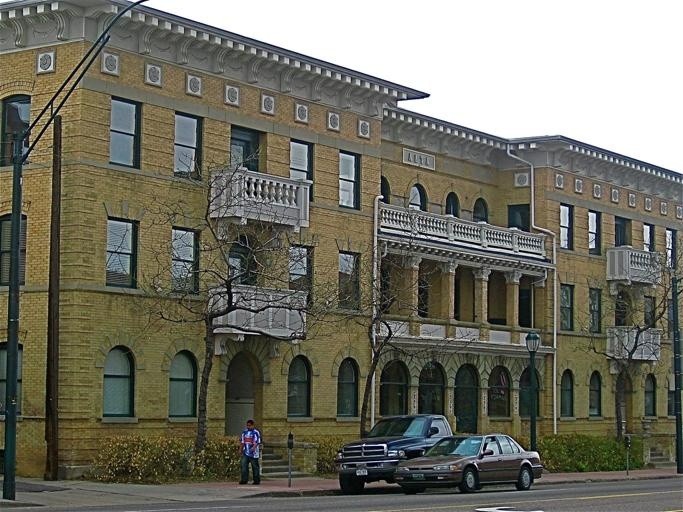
[393,431,542,492]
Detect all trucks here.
[334,408,452,492]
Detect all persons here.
[238,420,262,485]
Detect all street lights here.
[525,331,545,449]
